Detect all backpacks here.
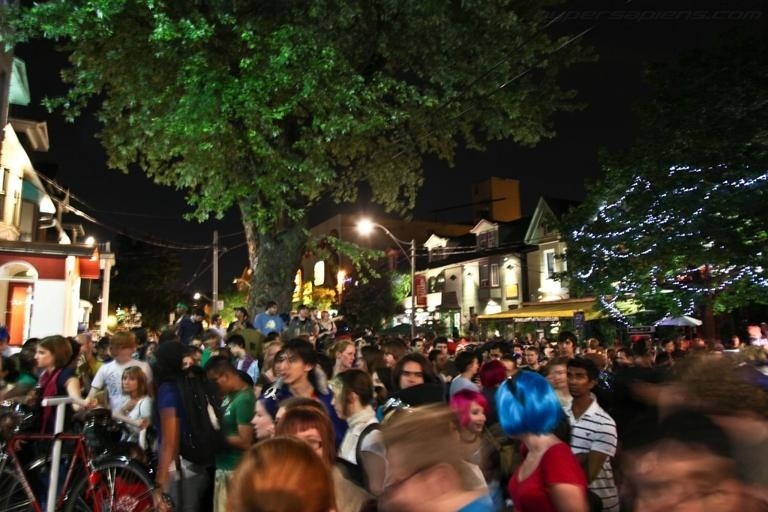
[177,365,225,465]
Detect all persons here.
[0,301,620,512]
[619,332,766,512]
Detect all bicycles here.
[0,397,176,511]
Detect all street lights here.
[358,220,417,343]
[193,292,212,303]
